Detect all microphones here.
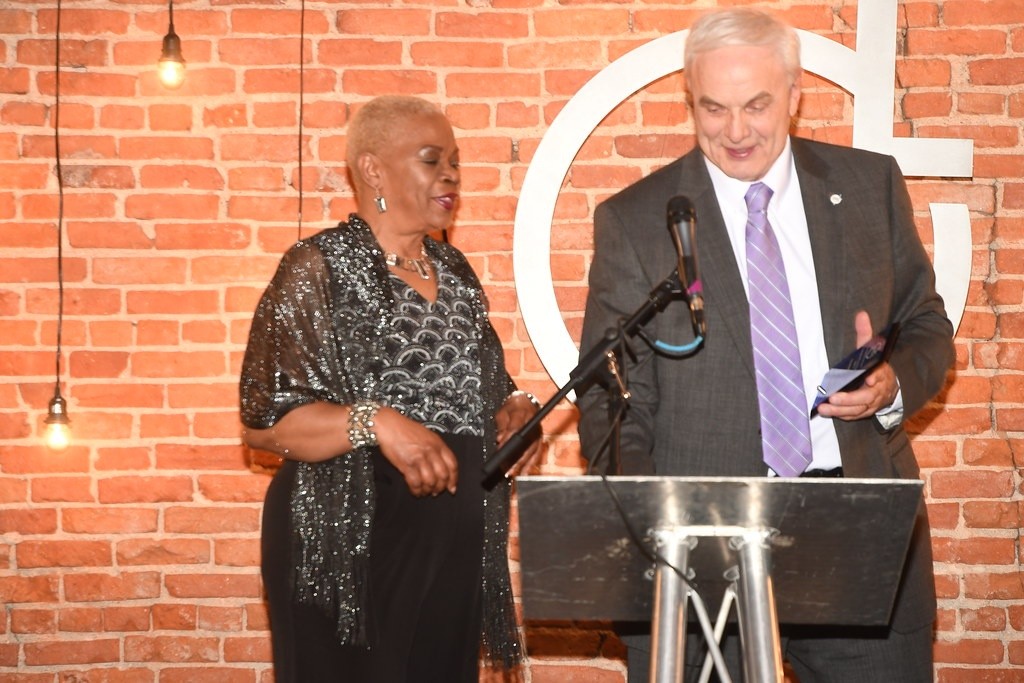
[668,194,706,340]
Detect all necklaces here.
[380,242,434,282]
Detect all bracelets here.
[347,398,377,447]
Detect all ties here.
[744,182,814,477]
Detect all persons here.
[239,95,537,682]
[578,7,957,683]
